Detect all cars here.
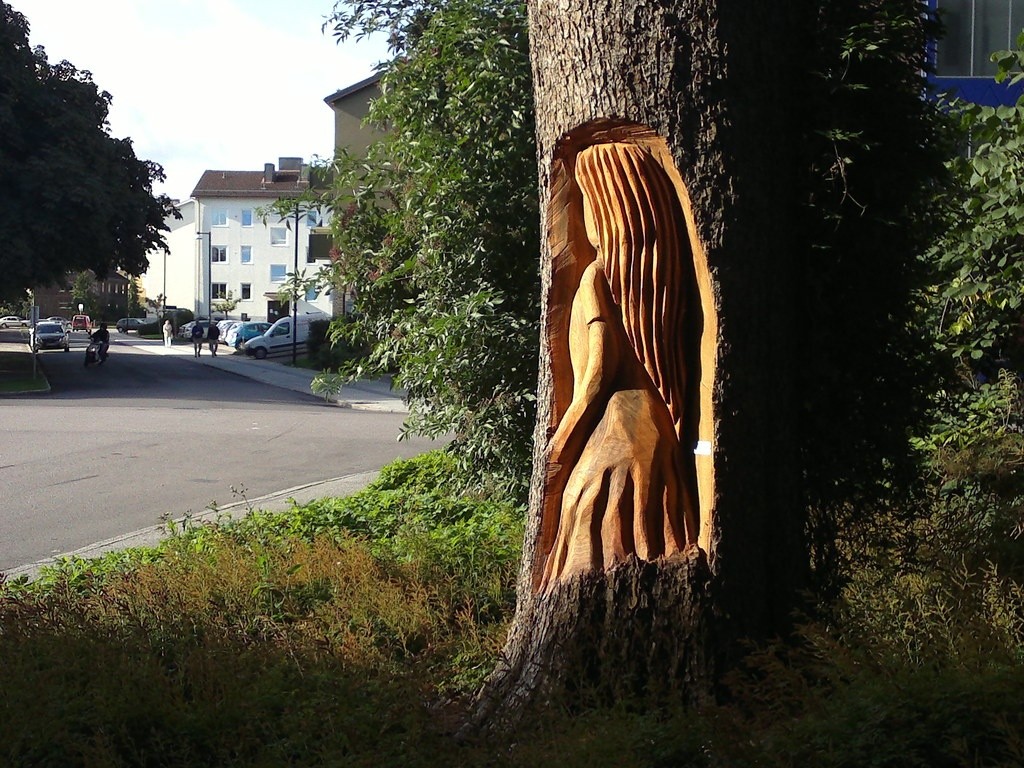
[235,322,288,351]
[115,317,149,333]
[0,316,31,328]
[38,317,72,328]
[29,322,71,353]
[177,319,252,347]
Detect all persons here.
[90,323,109,361]
[163,319,172,348]
[207,324,220,357]
[536,143,696,596]
[192,321,204,357]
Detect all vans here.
[243,313,331,359]
[72,315,93,332]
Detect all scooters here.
[83,326,103,368]
